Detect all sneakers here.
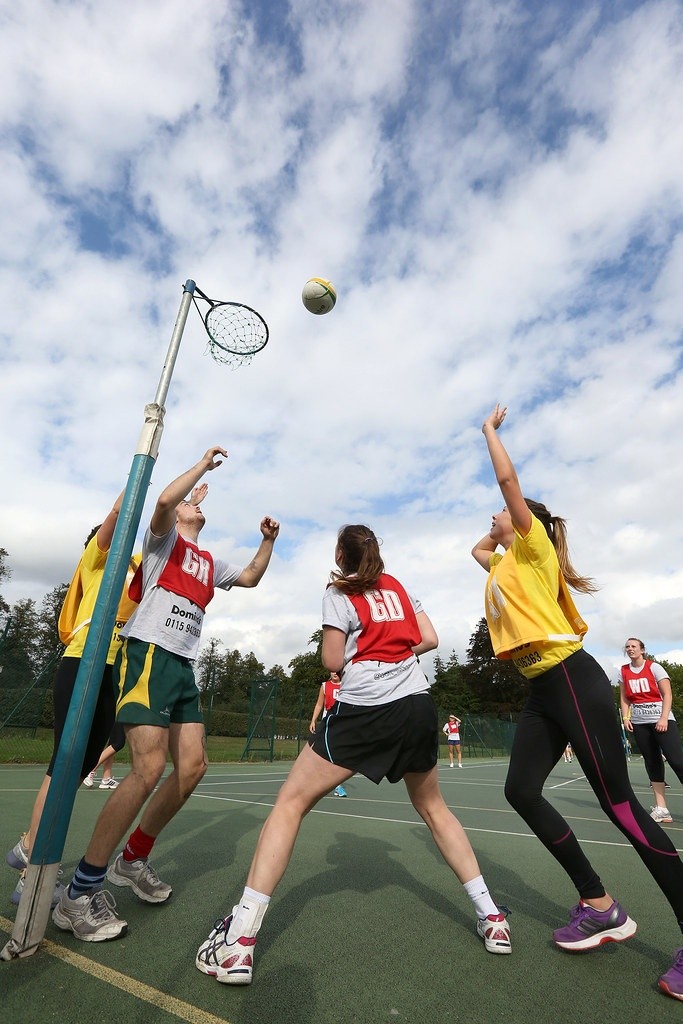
[83,772,97,787]
[648,805,673,823]
[657,948,683,1001]
[98,776,119,789]
[195,905,257,985]
[476,913,512,954]
[335,786,347,797]
[52,884,128,942]
[7,832,64,878]
[552,898,638,949]
[10,868,66,909]
[106,851,173,903]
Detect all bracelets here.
[622,717,629,720]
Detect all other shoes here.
[450,763,453,767]
[459,764,462,767]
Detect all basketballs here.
[301,277,337,316]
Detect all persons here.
[196,521,512,985]
[52,447,280,942]
[310,672,347,796]
[617,638,683,823]
[6,479,142,902]
[472,404,683,1000]
[83,724,127,788]
[562,742,573,763]
[443,715,462,769]
[624,738,632,758]
